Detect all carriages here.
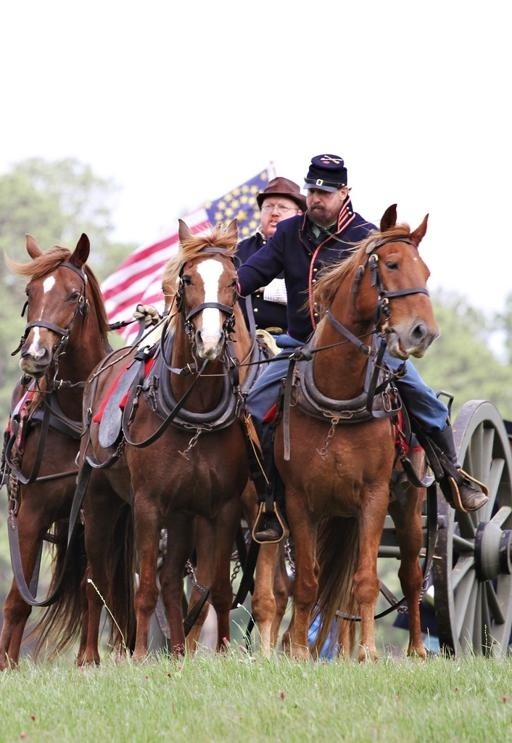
[0,203,512,671]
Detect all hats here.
[255,177,307,210]
[305,153,347,193]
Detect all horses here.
[76,219,253,672]
[255,203,435,672]
[3,234,138,668]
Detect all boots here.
[246,421,279,500]
[416,426,488,511]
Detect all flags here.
[97,163,279,346]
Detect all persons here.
[231,155,490,541]
[230,176,309,338]
